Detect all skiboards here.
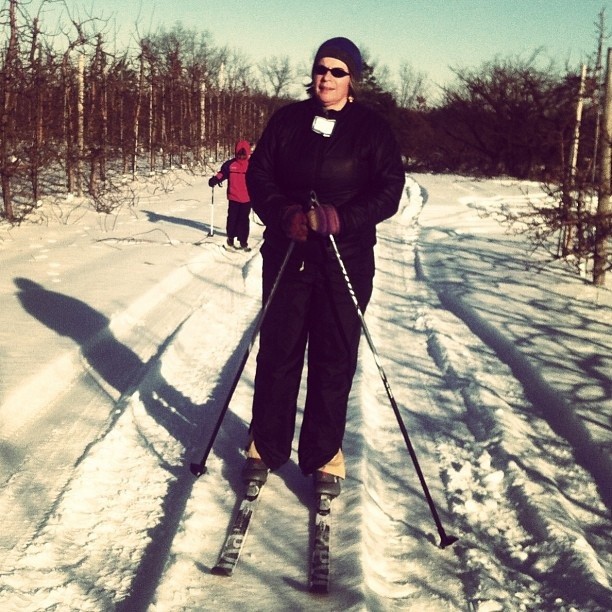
[210,483,334,595]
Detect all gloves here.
[209,176,218,186]
[280,204,307,244]
[308,203,341,236]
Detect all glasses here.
[314,65,350,77]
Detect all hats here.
[314,38,362,75]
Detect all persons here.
[245,37,405,497]
[209,140,252,248]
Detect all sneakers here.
[313,471,340,496]
[241,240,248,247]
[242,458,269,483]
[227,236,233,245]
